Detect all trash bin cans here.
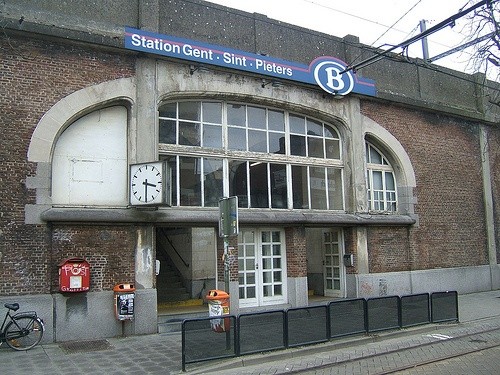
[206,288,231,333]
[114,283,135,322]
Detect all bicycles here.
[0,303,45,351]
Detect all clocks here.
[127,161,172,211]
